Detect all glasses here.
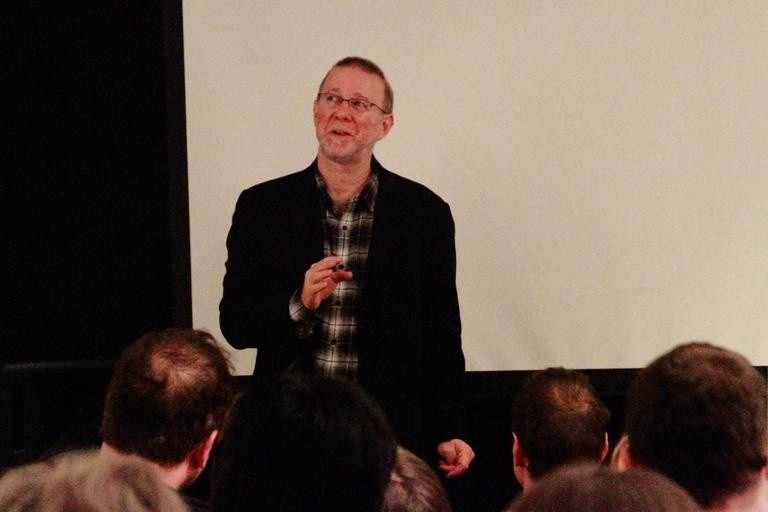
[317,93,385,113]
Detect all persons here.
[218,56,476,477]
[504,341,767,511]
[2,329,449,510]
